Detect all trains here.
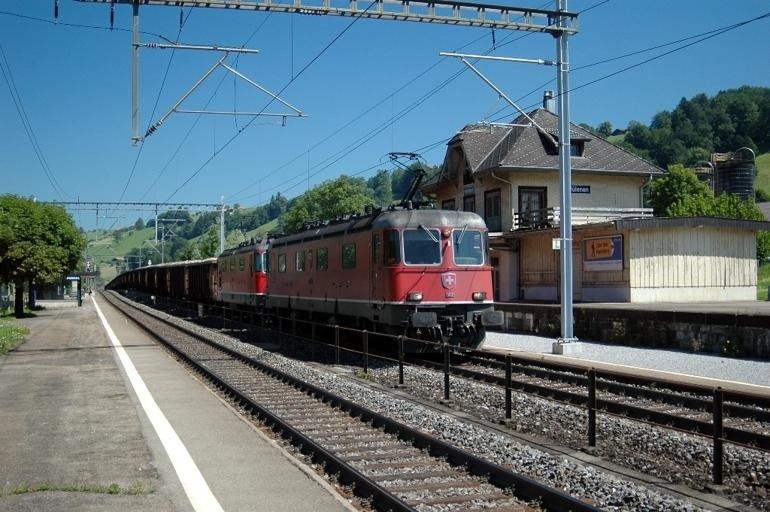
[103,202,496,363]
